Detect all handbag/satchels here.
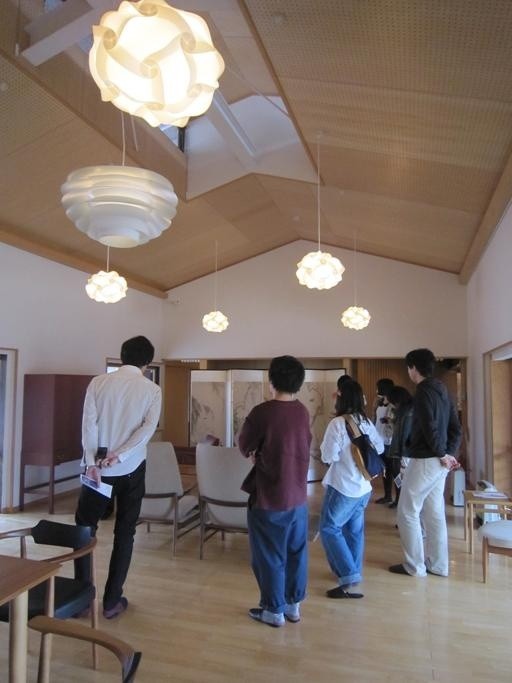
[350,434,386,482]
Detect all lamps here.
[202,240,229,332]
[341,229,371,330]
[85,246,128,303]
[88,0,226,128]
[60,111,179,248]
[295,135,345,290]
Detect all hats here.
[337,375,364,400]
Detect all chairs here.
[27,615,141,683]
[0,520,99,670]
[114,441,201,557]
[195,435,255,560]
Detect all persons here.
[386,386,416,508]
[74,336,163,616]
[238,356,312,628]
[372,378,397,505]
[388,349,463,578]
[318,375,386,598]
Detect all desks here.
[462,490,512,554]
[0,556,63,683]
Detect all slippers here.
[102,596,128,619]
[375,498,391,504]
[328,586,362,599]
[388,564,409,575]
[250,609,279,625]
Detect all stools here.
[478,520,512,583]
